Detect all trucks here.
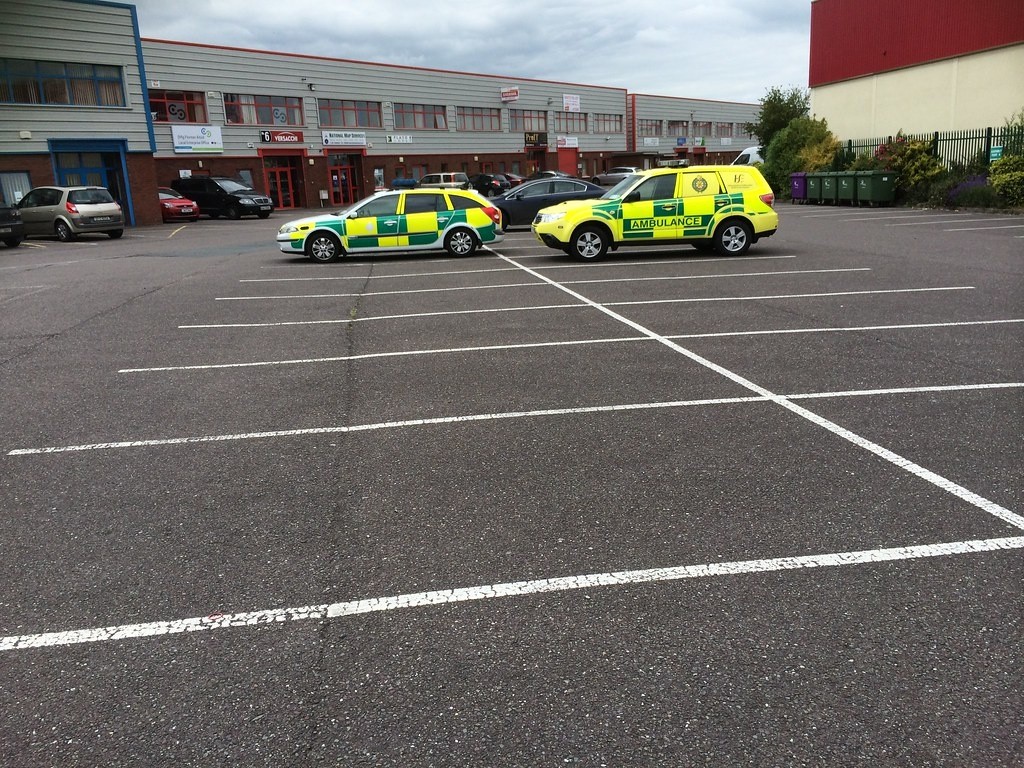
[729,145,765,165]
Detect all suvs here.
[9,183,125,242]
[171,174,275,220]
[530,158,780,263]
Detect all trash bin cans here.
[790,170,897,208]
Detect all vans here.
[417,171,474,189]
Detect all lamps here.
[310,84,318,91]
[548,98,554,104]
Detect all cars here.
[498,172,527,190]
[157,185,200,224]
[0,198,26,247]
[468,172,511,198]
[275,177,505,264]
[489,176,609,231]
[590,166,643,186]
[528,169,577,180]
